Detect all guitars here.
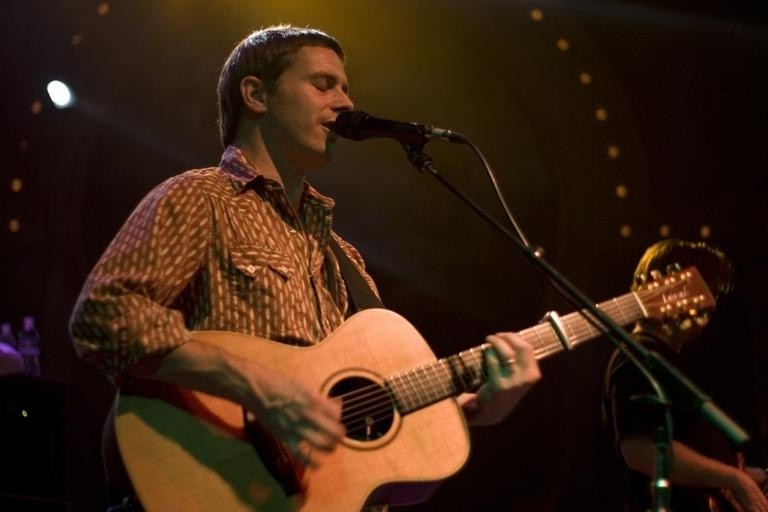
[100,263,717,512]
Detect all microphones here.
[335,110,464,151]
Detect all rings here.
[501,358,519,366]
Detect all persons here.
[69,27,541,512]
[604,241,768,511]
